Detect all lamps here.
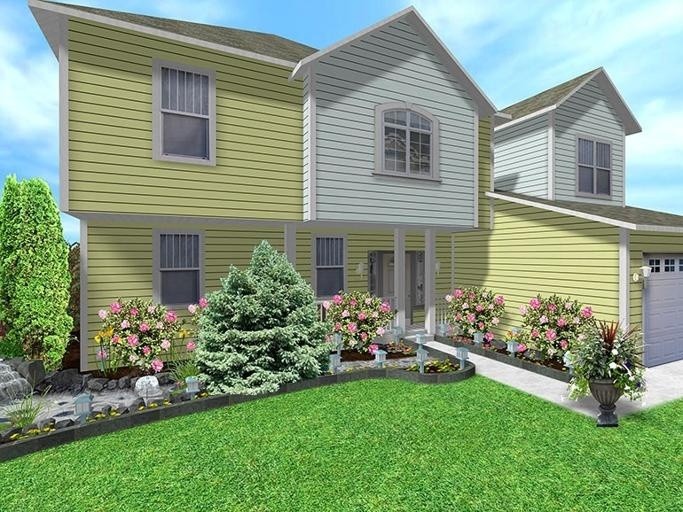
[329,354,343,375]
[440,323,448,336]
[505,340,518,358]
[358,262,365,280]
[72,391,93,424]
[456,347,469,370]
[415,333,426,348]
[392,327,403,345]
[415,348,429,374]
[472,332,483,346]
[633,265,653,291]
[185,375,200,401]
[373,349,387,369]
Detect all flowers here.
[562,315,648,402]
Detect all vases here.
[588,380,624,428]
